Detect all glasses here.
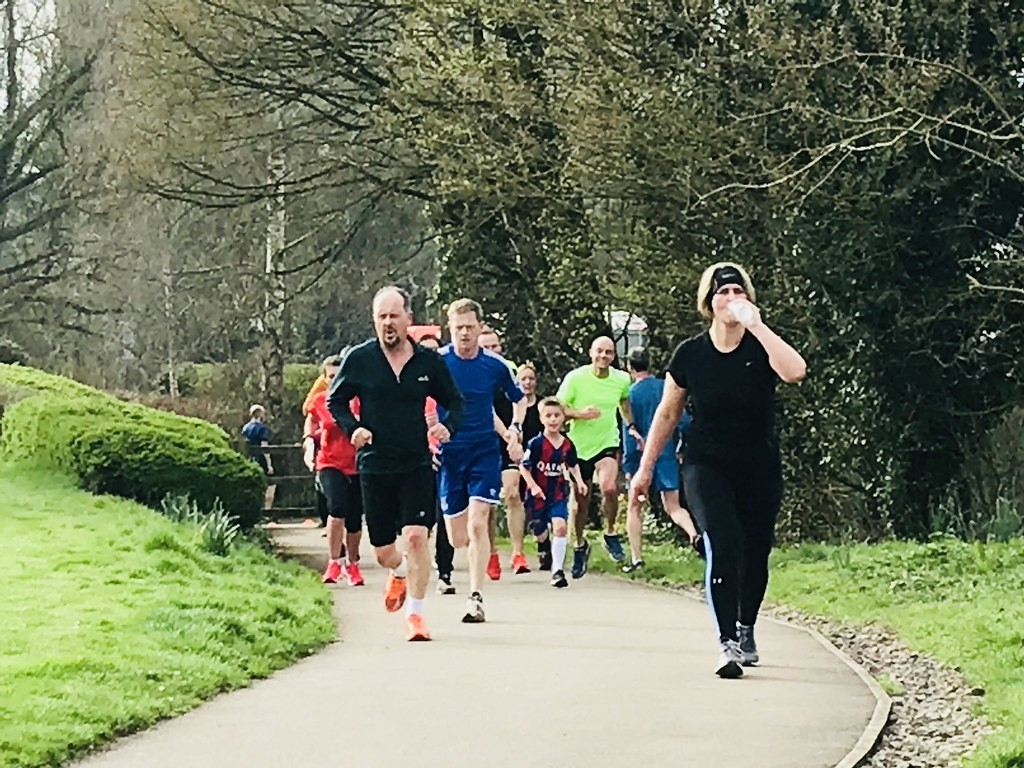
[715,287,747,295]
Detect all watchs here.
[514,422,522,432]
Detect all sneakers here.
[552,569,569,588]
[715,643,743,678]
[341,561,364,587]
[736,622,760,667]
[512,554,531,574]
[604,534,625,562]
[571,539,591,580]
[461,590,485,623]
[322,527,327,537]
[691,534,707,560]
[538,540,552,571]
[621,559,645,574]
[322,561,341,582]
[487,554,501,580]
[340,543,347,557]
[385,575,406,613]
[406,614,431,641]
[437,576,456,595]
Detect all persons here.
[417,334,456,594]
[519,396,588,587]
[630,262,807,677]
[431,298,528,624]
[621,346,706,573]
[477,329,531,580]
[515,364,552,571]
[241,405,277,523]
[556,336,645,579]
[302,345,365,587]
[325,285,465,641]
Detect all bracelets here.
[302,434,315,443]
[625,422,635,431]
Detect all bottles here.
[729,301,754,324]
